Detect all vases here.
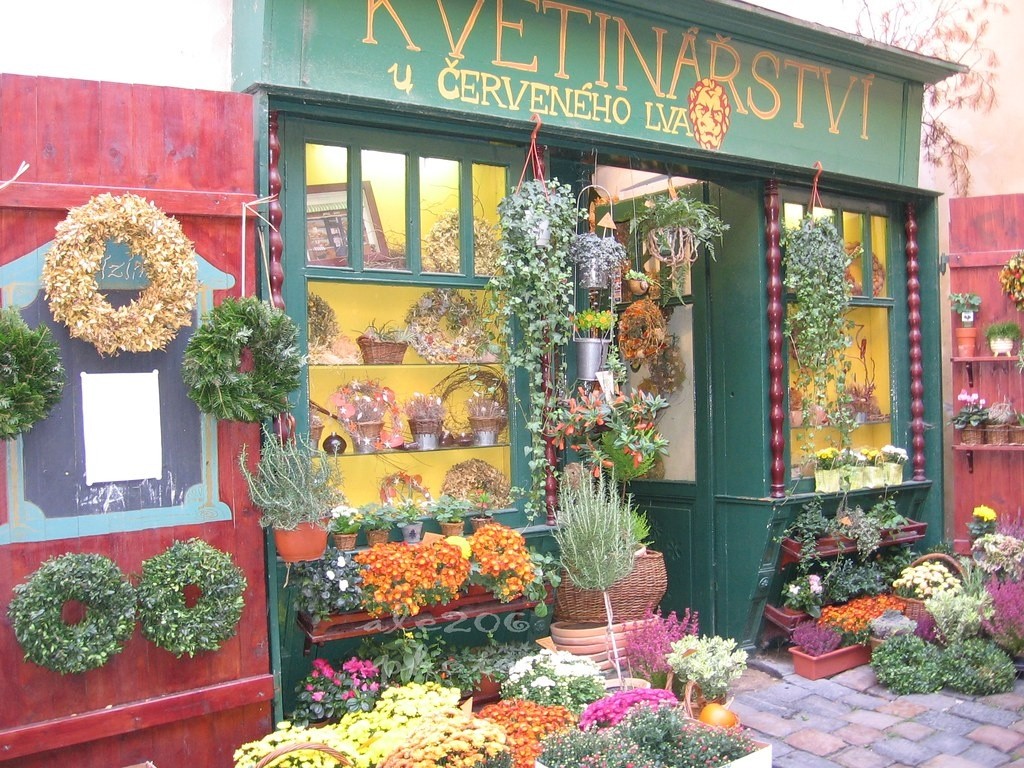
[782,604,804,617]
[881,462,905,486]
[962,425,984,445]
[399,522,423,543]
[348,421,385,453]
[814,469,841,494]
[467,416,502,446]
[985,394,1012,425]
[870,637,890,654]
[301,714,332,729]
[788,640,872,681]
[574,338,611,381]
[843,466,863,490]
[408,419,444,450]
[307,425,324,449]
[862,466,884,488]
[956,328,977,358]
[987,425,1009,446]
[467,583,489,597]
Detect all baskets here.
[961,423,1024,445]
[439,520,465,537]
[356,335,409,364]
[471,515,493,534]
[892,553,973,636]
[665,649,744,735]
[408,419,443,434]
[309,425,325,439]
[552,549,667,623]
[467,416,507,432]
[331,532,359,551]
[365,530,389,547]
[355,421,384,436]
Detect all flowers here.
[405,392,447,418]
[324,506,360,534]
[290,659,358,718]
[573,309,617,339]
[948,389,988,428]
[465,523,536,605]
[333,379,388,421]
[308,403,324,427]
[395,499,429,527]
[465,390,508,417]
[781,445,916,657]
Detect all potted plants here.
[1011,411,1024,444]
[783,493,928,554]
[237,415,348,564]
[360,503,403,550]
[459,639,537,696]
[789,387,806,426]
[845,336,875,422]
[575,235,628,289]
[426,495,471,535]
[600,508,656,555]
[624,269,653,295]
[984,322,1019,357]
[630,192,730,280]
[471,491,497,532]
[949,291,981,327]
